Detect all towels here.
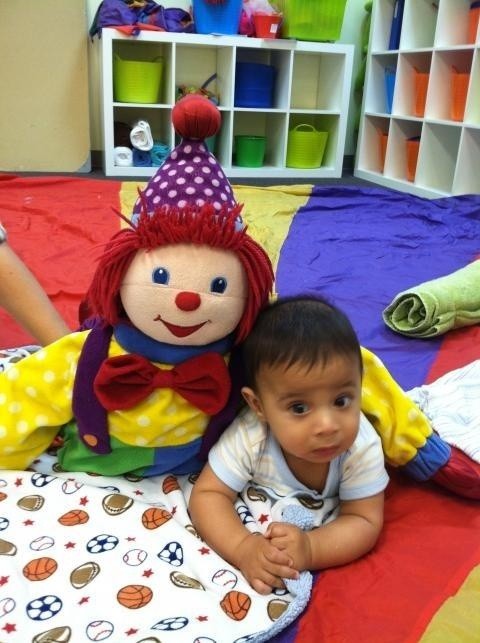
[382,255,480,340]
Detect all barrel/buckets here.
[384,66,396,115]
[234,62,277,107]
[112,52,165,103]
[234,134,270,168]
[375,128,388,173]
[287,124,329,168]
[410,65,429,117]
[404,135,421,181]
[449,65,470,122]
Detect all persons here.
[1,92,480,502]
[1,211,75,351]
[187,289,393,596]
